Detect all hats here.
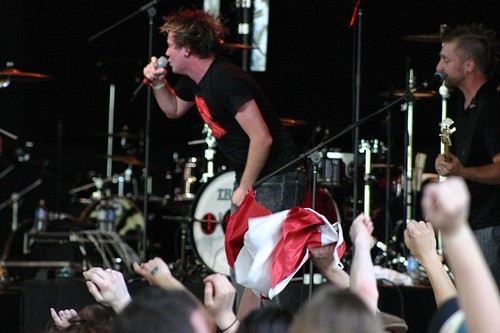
[438,310,468,333]
[377,312,408,332]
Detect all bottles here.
[97,200,107,231]
[108,201,114,231]
[408,251,420,285]
[35,200,47,232]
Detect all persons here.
[434,23,500,291]
[0,176,500,333]
[143,10,306,214]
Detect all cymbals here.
[0,68,48,79]
[103,154,146,172]
[388,89,439,99]
[405,32,454,41]
[109,130,142,139]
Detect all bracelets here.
[154,83,165,90]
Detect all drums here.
[187,172,343,278]
[74,195,151,269]
[302,151,359,189]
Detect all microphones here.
[350,0,361,26]
[133,56,168,95]
[421,70,447,89]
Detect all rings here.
[439,168,441,170]
